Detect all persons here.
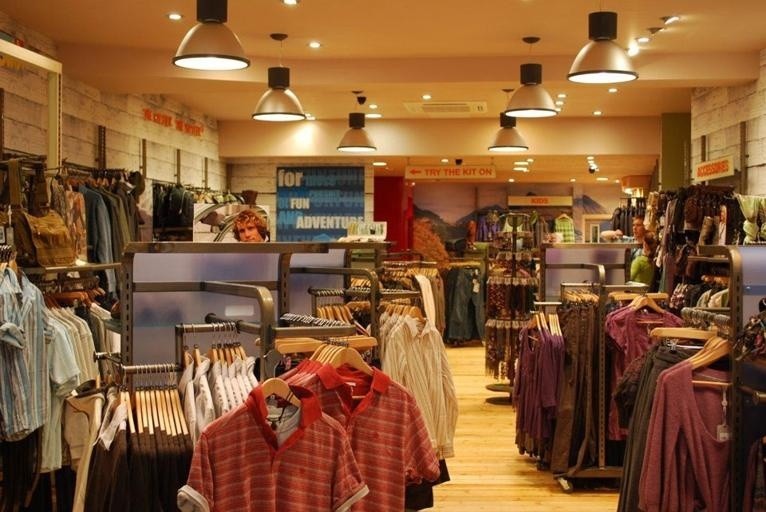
[601,215,645,259]
[719,204,728,245]
[233,209,265,243]
[630,232,659,284]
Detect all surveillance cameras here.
[590,168,595,173]
[456,159,463,165]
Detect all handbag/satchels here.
[8,207,78,270]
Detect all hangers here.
[518,274,766,392]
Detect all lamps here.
[334,88,378,154]
[252,29,307,123]
[484,0,638,154]
[170,0,252,72]
